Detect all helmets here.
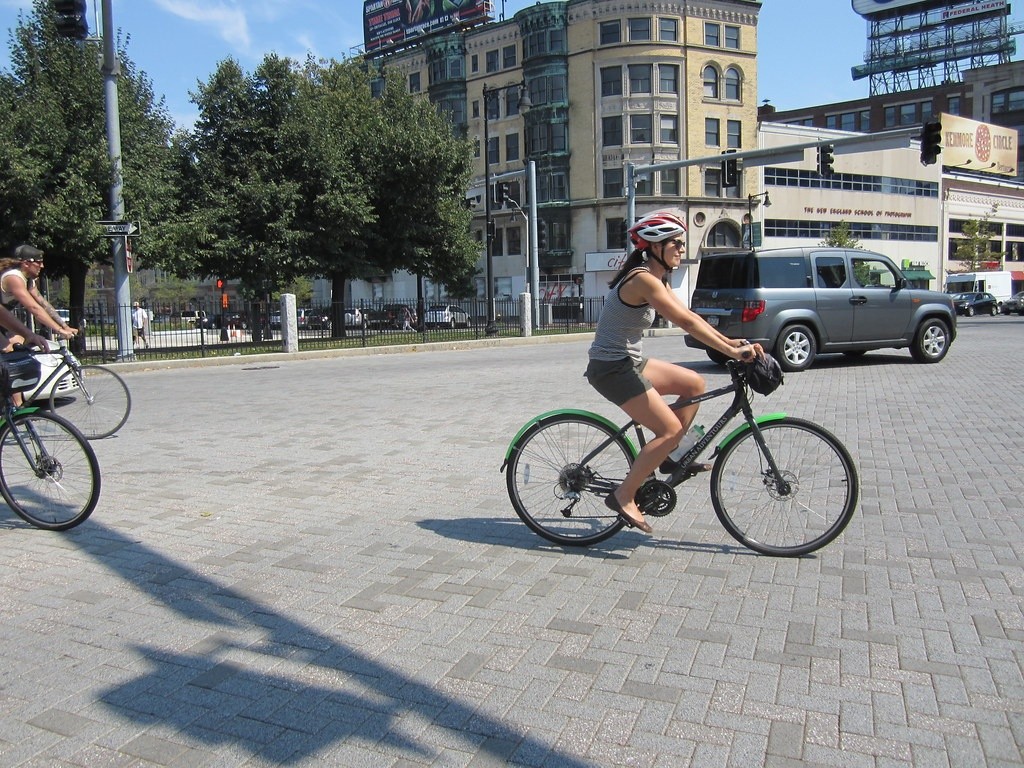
[627,211,687,251]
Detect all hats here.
[15,245,44,261]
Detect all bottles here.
[664,424,706,461]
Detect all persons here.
[131,302,148,348]
[0,244,78,407]
[583,212,765,534]
[0,305,51,353]
[401,306,414,332]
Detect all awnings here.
[902,270,936,281]
[1010,271,1024,281]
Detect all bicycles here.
[0,330,131,441]
[0,340,102,532]
[500,340,861,560]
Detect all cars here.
[424,303,473,329]
[16,334,86,405]
[951,291,998,317]
[179,301,420,332]
[55,308,70,326]
[1001,290,1024,316]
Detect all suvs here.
[681,245,959,374]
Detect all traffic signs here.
[95,220,142,237]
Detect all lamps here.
[982,163,996,169]
[949,160,971,168]
[1000,168,1015,173]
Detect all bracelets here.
[58,327,62,332]
[65,326,69,328]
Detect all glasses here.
[667,238,685,250]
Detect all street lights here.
[748,189,772,250]
[482,69,535,338]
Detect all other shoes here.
[604,493,652,533]
[659,459,711,474]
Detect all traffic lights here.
[54,0,88,38]
[217,279,223,288]
[816,143,835,175]
[497,183,509,203]
[921,120,942,164]
